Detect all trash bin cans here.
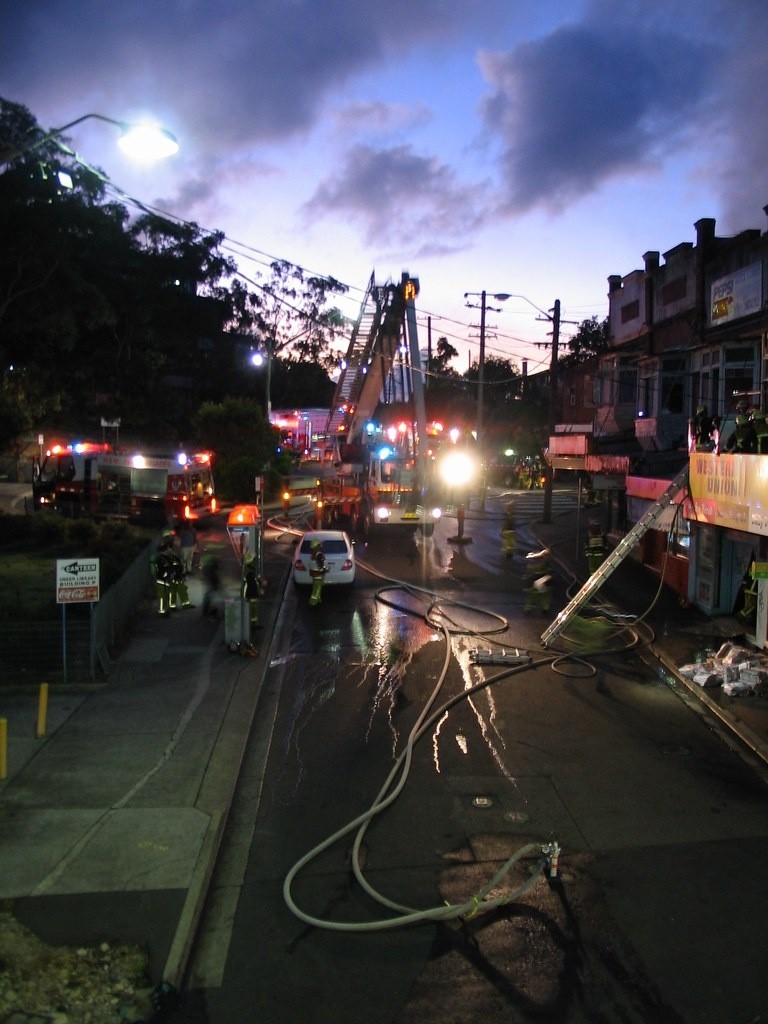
[224,600,250,652]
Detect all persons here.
[167,540,195,610]
[516,454,544,489]
[155,543,174,618]
[177,520,199,576]
[243,553,264,629]
[308,538,331,605]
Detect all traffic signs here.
[55,558,100,604]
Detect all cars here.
[292,531,356,584]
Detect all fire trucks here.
[270,269,473,544]
[33,443,222,529]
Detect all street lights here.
[465,290,510,507]
[252,338,272,415]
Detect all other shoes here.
[157,613,169,618]
[169,608,178,611]
[186,571,193,575]
[183,605,196,609]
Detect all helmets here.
[735,414,747,427]
[751,410,765,420]
[310,538,320,548]
[735,400,747,409]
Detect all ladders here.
[468,649,533,664]
[539,463,690,650]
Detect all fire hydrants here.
[549,841,561,877]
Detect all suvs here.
[486,454,545,488]
